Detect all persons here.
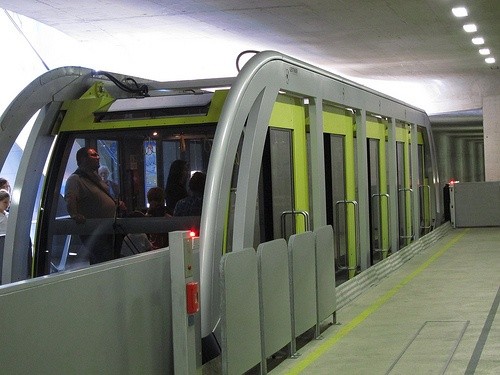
[145,158,206,216]
[120,210,158,257]
[99,166,119,201]
[64,147,124,266]
[0,177,14,236]
